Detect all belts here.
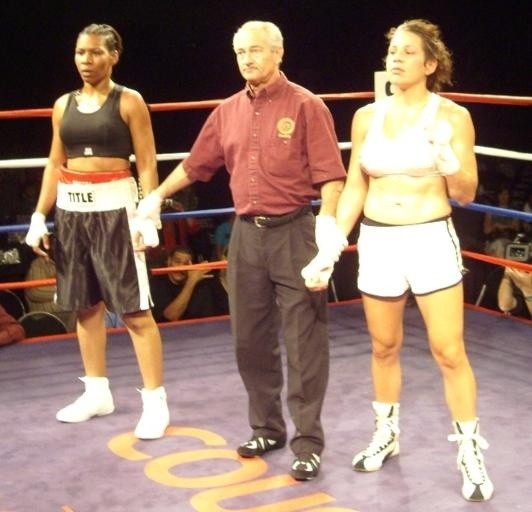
[240,204,312,228]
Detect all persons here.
[22,21,172,441]
[131,19,347,485]
[1,172,532,346]
[297,19,496,504]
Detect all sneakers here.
[292,453,320,480]
[237,436,285,458]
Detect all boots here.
[351,401,401,472]
[447,418,494,501]
[135,386,170,440]
[56,376,115,423]
